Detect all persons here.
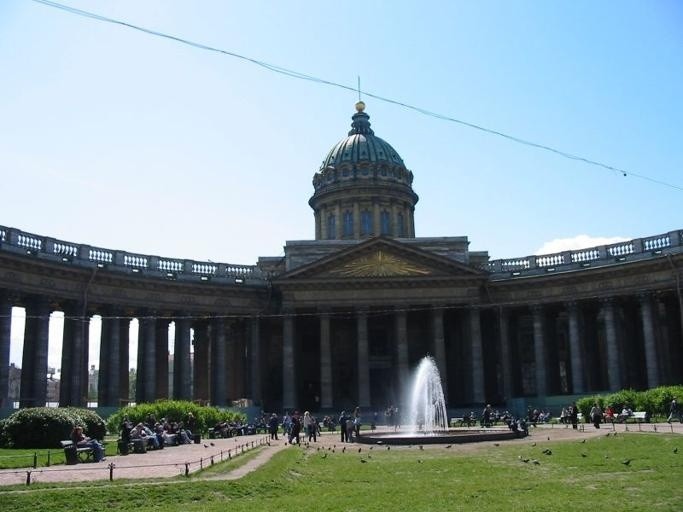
[559,402,578,426]
[667,397,682,423]
[120,412,195,454]
[268,413,280,440]
[618,405,633,424]
[469,412,477,427]
[479,403,551,430]
[604,406,615,422]
[282,405,401,444]
[590,404,601,429]
[211,421,267,440]
[72,426,106,462]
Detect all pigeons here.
[518,454,541,467]
[317,444,392,463]
[582,440,586,442]
[542,449,552,455]
[623,459,631,464]
[581,454,586,457]
[674,448,677,452]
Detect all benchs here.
[555,411,647,423]
[60,440,95,464]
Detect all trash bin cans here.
[64,444,78,465]
[194,435,200,444]
[209,428,215,439]
